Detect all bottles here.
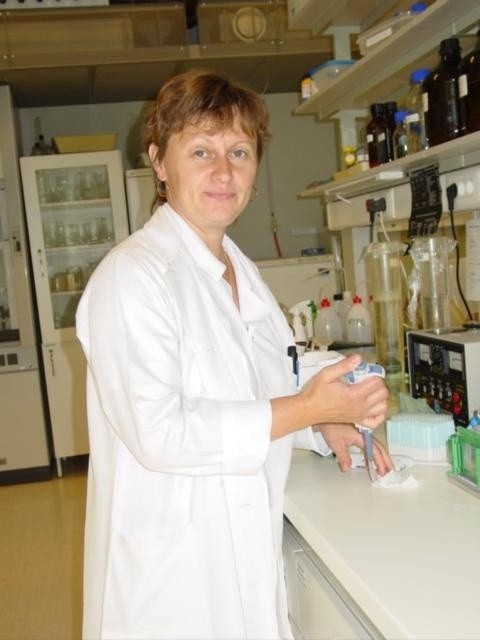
[32,134,53,156]
[368,238,450,393]
[315,293,375,346]
[40,171,114,292]
[302,74,314,104]
[344,29,479,169]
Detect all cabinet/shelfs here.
[289,1,480,202]
[0,84,131,477]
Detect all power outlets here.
[365,189,396,223]
[443,166,480,212]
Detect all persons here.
[75,68,396,640]
